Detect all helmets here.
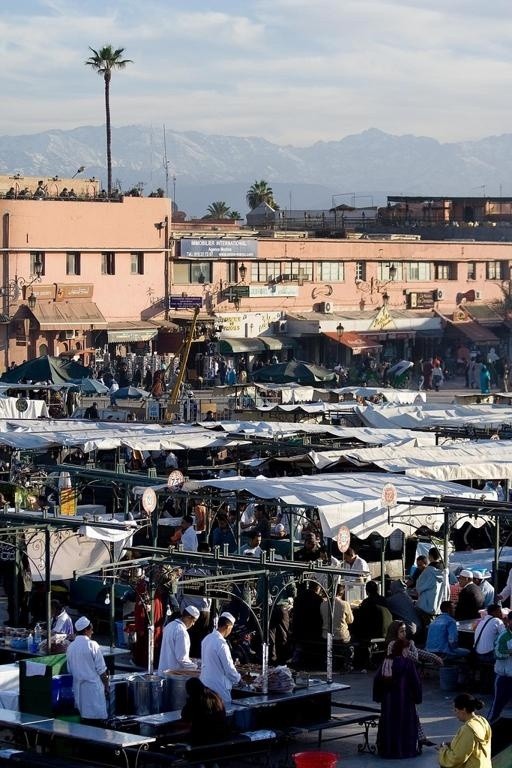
[46,597,64,614]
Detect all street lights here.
[336,323,344,365]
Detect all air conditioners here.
[321,302,334,314]
[275,319,288,333]
[475,291,482,300]
[65,330,76,340]
[435,288,444,301]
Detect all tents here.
[186,472,498,543]
[355,400,512,437]
[37,431,251,469]
[244,382,331,403]
[323,386,424,409]
[192,422,415,456]
[255,401,358,427]
[335,382,426,404]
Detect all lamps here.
[380,264,397,289]
[26,287,37,309]
[236,264,247,286]
[21,259,43,287]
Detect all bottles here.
[27,633,33,651]
[35,622,42,649]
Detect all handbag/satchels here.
[372,660,391,700]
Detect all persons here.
[486,612,512,722]
[416,547,458,622]
[183,391,197,423]
[483,480,505,501]
[372,620,443,750]
[235,532,263,558]
[473,570,495,606]
[293,581,324,642]
[350,581,392,670]
[84,402,100,419]
[177,516,198,551]
[463,356,492,394]
[200,612,245,706]
[256,555,293,666]
[474,604,506,660]
[166,450,179,471]
[66,616,110,728]
[83,360,183,401]
[435,693,493,768]
[221,588,256,666]
[177,557,212,640]
[155,578,180,668]
[340,549,371,602]
[416,355,445,391]
[202,411,216,422]
[107,375,120,406]
[227,510,237,524]
[497,566,511,609]
[276,524,291,540]
[373,639,424,760]
[406,555,428,588]
[158,605,199,679]
[37,599,74,641]
[216,358,265,388]
[425,602,471,664]
[212,503,230,528]
[128,413,137,420]
[294,533,322,561]
[220,456,237,477]
[313,545,341,588]
[385,580,419,631]
[456,570,485,620]
[211,514,236,553]
[180,678,228,744]
[321,584,354,643]
[242,505,272,539]
[135,578,161,666]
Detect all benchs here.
[331,695,383,713]
[293,711,381,756]
[157,725,304,768]
[1,742,112,767]
[415,655,468,687]
[368,639,387,671]
[331,698,383,713]
[276,638,361,673]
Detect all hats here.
[76,615,93,633]
[275,523,284,532]
[458,568,471,579]
[218,611,237,625]
[474,572,482,581]
[186,604,201,618]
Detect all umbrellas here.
[251,357,340,385]
[64,377,109,395]
[2,355,93,385]
[111,385,151,408]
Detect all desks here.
[231,681,351,727]
[21,718,157,767]
[0,628,131,663]
[426,617,480,634]
[132,702,248,738]
[0,709,55,752]
